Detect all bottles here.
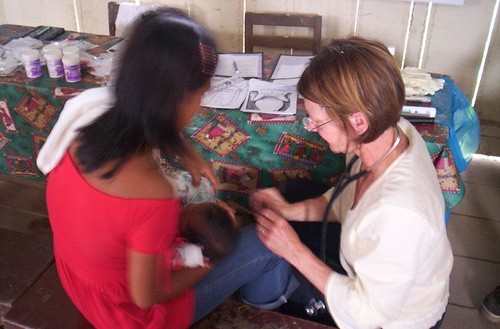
[22,48,43,79]
[42,43,82,83]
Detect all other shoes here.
[480,285,500,323]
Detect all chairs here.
[244,12,323,56]
[109,1,160,36]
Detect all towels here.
[35,87,118,176]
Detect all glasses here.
[302,113,352,132]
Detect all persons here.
[152,146,238,262]
[38,5,324,329]
[247,36,455,329]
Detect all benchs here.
[189,298,336,329]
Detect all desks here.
[0,24,482,232]
